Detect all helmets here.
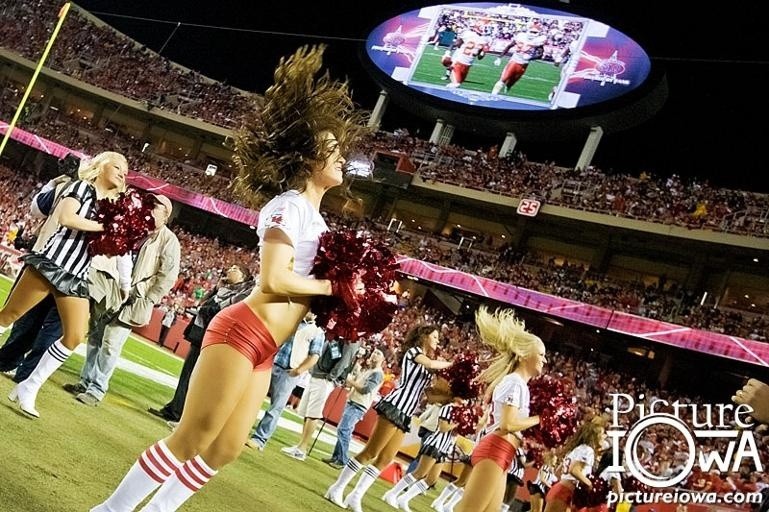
[469,21,542,38]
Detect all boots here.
[6,339,72,419]
[325,456,382,512]
[139,452,219,512]
[430,482,465,512]
[382,473,429,512]
[501,502,511,512]
[92,439,184,512]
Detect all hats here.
[145,193,174,217]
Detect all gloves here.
[442,50,531,68]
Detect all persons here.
[0,0,769,512]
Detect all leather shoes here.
[147,404,176,420]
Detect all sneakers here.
[63,380,103,407]
[246,435,265,452]
[279,445,306,463]
[321,456,345,470]
